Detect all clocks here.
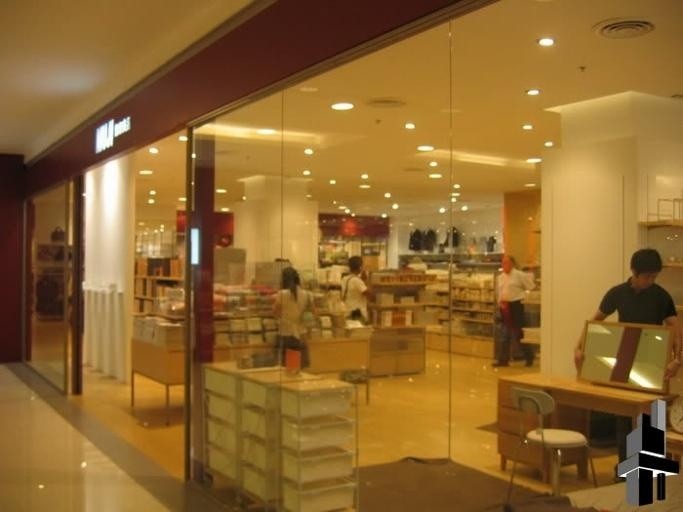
[668,391,682,435]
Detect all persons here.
[342,255,370,323]
[272,265,320,373]
[574,248,682,485]
[490,253,537,369]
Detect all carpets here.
[350,455,600,509]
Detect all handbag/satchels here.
[50,225,67,242]
[38,246,54,262]
[56,247,73,262]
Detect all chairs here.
[500,385,601,503]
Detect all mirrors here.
[572,317,675,394]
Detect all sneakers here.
[490,358,509,366]
[524,349,535,367]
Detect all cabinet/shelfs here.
[640,215,682,272]
[195,355,363,511]
[504,189,543,269]
[31,242,75,320]
[130,247,537,424]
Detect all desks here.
[496,370,666,474]
